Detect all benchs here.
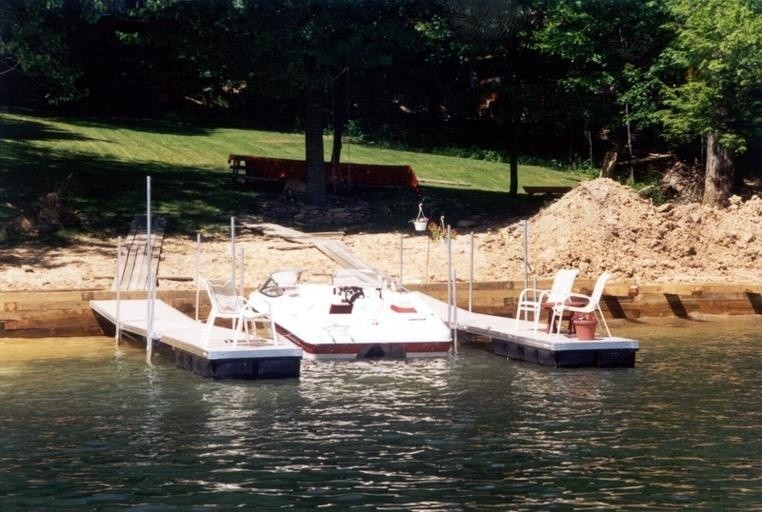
[523,185,572,212]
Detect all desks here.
[228,153,422,196]
[540,301,587,337]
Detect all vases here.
[413,221,427,231]
[572,318,598,341]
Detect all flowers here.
[408,217,429,223]
[428,222,457,241]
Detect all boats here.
[247,267,453,362]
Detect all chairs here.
[546,272,613,339]
[197,280,278,347]
[513,269,579,334]
